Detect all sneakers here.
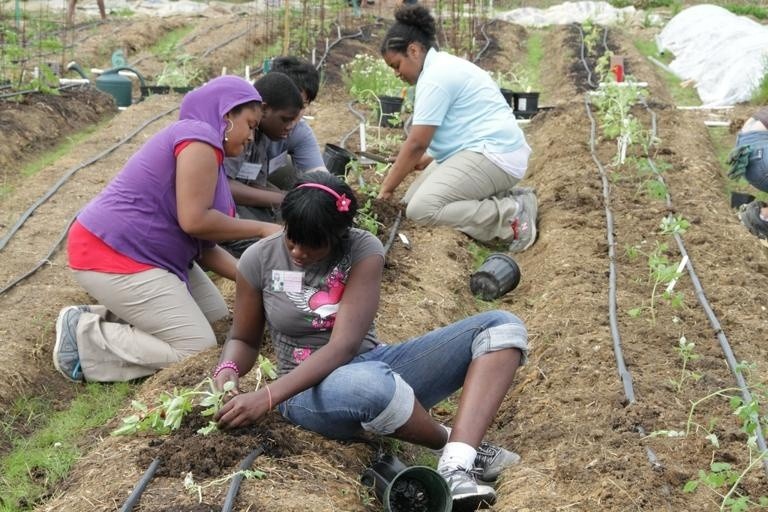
[738,201,768,240]
[436,442,521,512]
[52,305,90,383]
[508,185,538,254]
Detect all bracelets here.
[266,386,272,412]
[213,361,239,377]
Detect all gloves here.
[724,143,751,180]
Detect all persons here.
[723,105,768,241]
[51,75,289,385]
[211,170,528,509]
[218,71,304,262]
[261,54,329,206]
[374,3,538,255]
